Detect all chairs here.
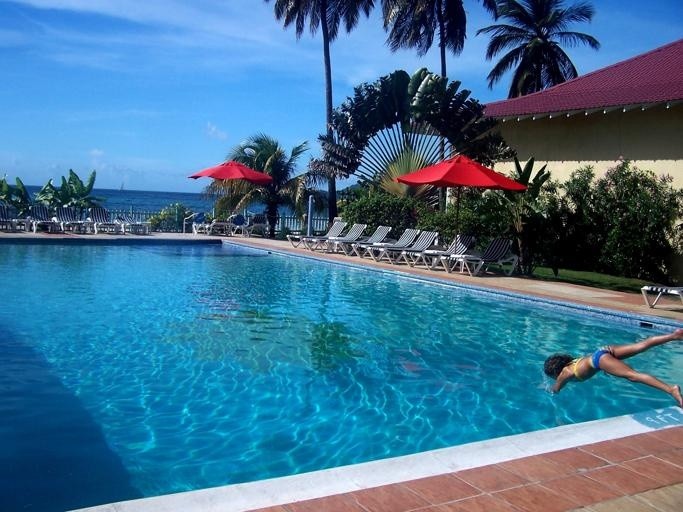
[0,205,152,235]
[284,218,518,279]
[183,212,269,240]
[640,285,683,310]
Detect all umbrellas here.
[395,152,532,254]
[188,159,274,234]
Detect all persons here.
[542,325,683,408]
